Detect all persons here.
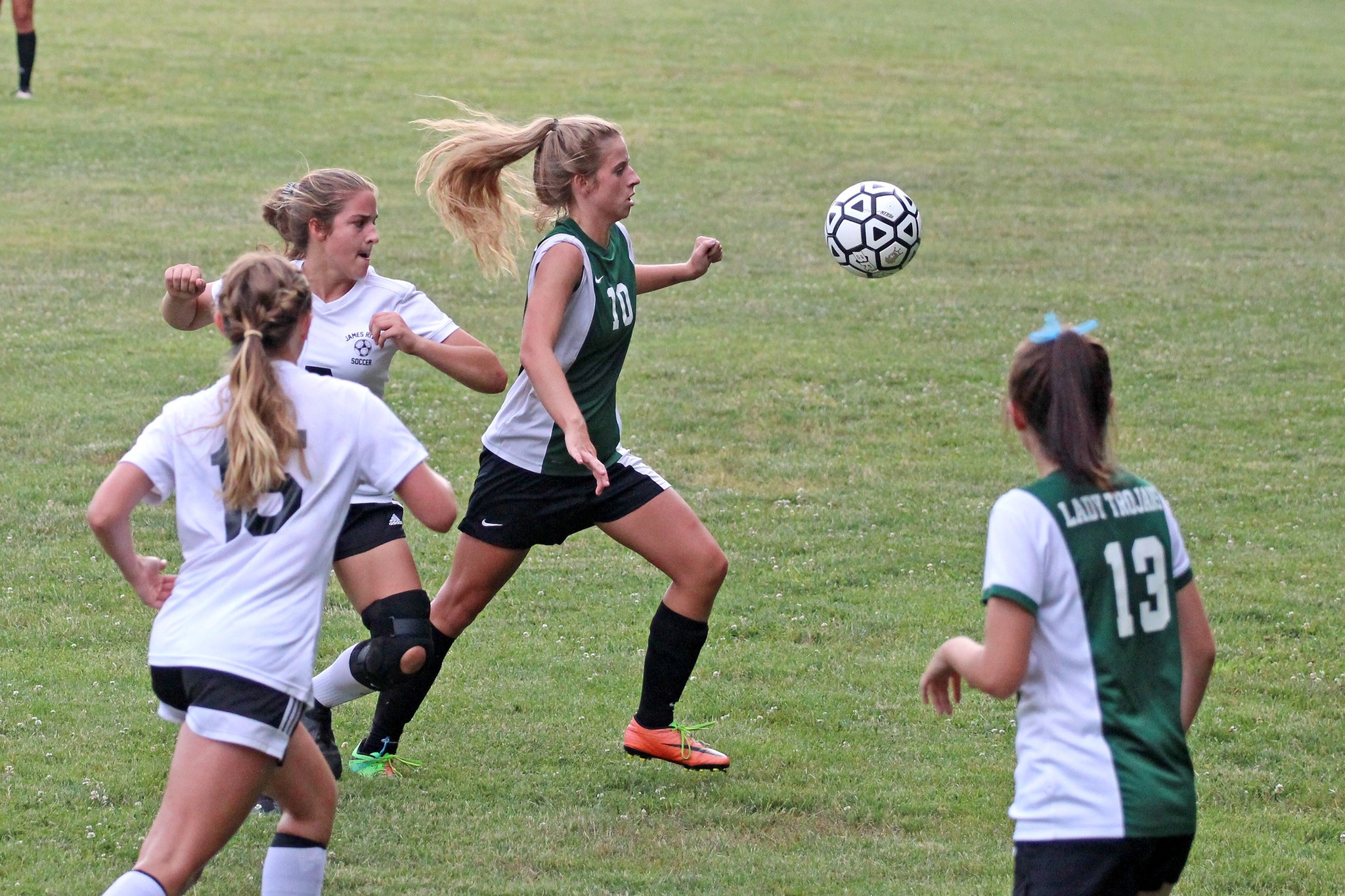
[93,251,459,896]
[337,93,738,783]
[150,167,552,770]
[921,314,1218,896]
[10,0,37,102]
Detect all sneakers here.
[622,714,731,772]
[300,701,344,780]
[254,795,280,820]
[349,736,424,783]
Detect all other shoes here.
[16,88,31,99]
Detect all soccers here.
[824,180,922,279]
